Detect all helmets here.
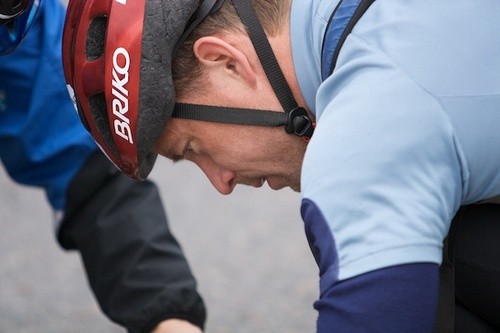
[61,0,219,180]
[0,0,42,55]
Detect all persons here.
[59,0,500,332]
[0,0,206,332]
